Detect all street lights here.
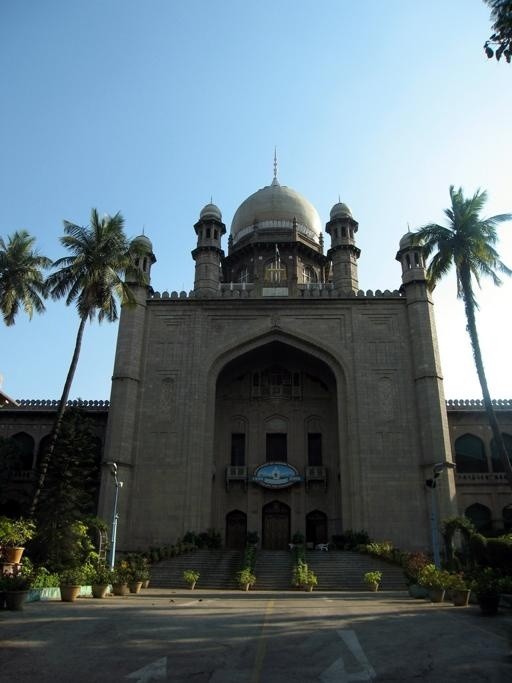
[106,461,124,573]
[429,462,445,570]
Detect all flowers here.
[0,563,35,591]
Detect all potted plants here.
[0,516,39,563]
[59,560,153,601]
[363,571,384,591]
[408,557,501,616]
[236,574,256,591]
[184,569,201,589]
[296,575,318,592]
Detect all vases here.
[5,592,29,611]
